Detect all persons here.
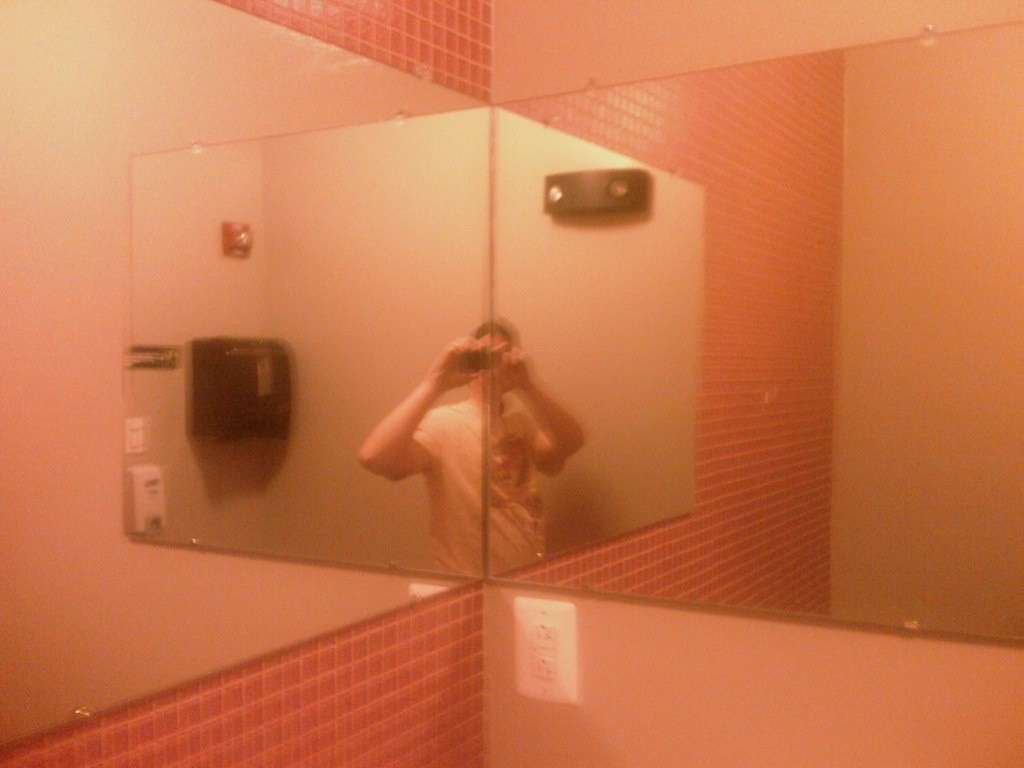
[354,318,583,579]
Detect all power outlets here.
[512,596,577,703]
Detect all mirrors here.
[494,105,706,572]
[1,1,489,750]
[118,110,485,585]
[492,18,1024,641]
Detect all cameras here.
[458,348,500,371]
